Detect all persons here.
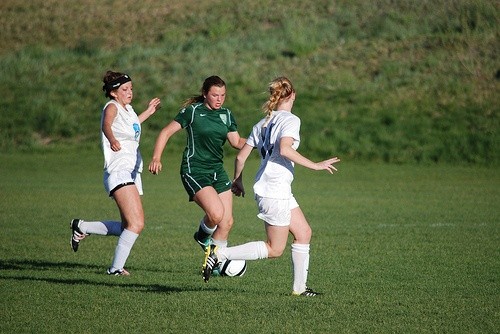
[69,70,161,277]
[201,77,341,297]
[148,75,247,277]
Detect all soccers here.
[218,259,247,277]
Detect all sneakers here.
[69,218,91,252]
[201,244,222,283]
[292,288,324,297]
[193,232,207,252]
[106,268,130,276]
